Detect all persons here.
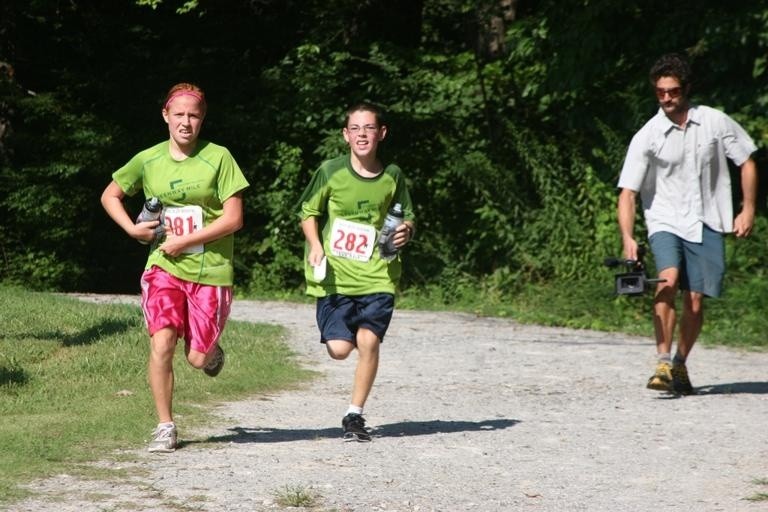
[99,81,252,452]
[294,101,419,443]
[614,55,760,394]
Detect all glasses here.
[656,86,684,100]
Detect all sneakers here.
[646,361,672,390]
[341,413,369,444]
[205,344,224,377]
[674,359,691,395]
[147,422,177,453]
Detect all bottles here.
[377,203,404,261]
[136,197,163,244]
